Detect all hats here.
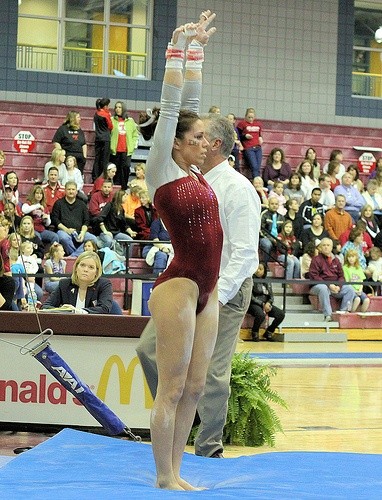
[3,185,12,191]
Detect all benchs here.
[0,100,379,315]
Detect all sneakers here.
[252,331,259,341]
[263,330,272,341]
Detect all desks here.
[0,311,154,441]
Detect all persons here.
[366,246,382,295]
[41,147,174,276]
[38,251,112,314]
[341,227,376,295]
[136,115,261,458]
[140,10,216,490]
[342,250,370,318]
[228,155,235,168]
[226,113,244,173]
[209,106,221,117]
[53,111,87,175]
[0,150,66,312]
[248,259,285,342]
[253,148,382,280]
[309,237,354,322]
[236,107,263,180]
[110,101,140,192]
[91,97,114,182]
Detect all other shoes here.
[210,450,224,458]
[326,315,332,321]
